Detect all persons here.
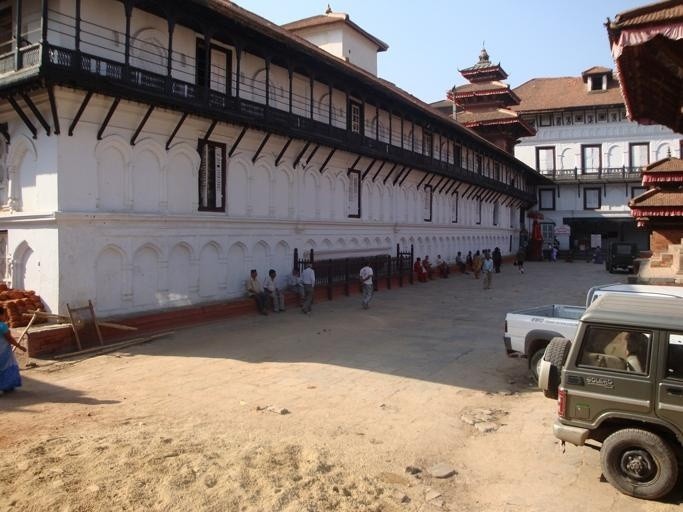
[594,245,602,264]
[545,235,560,262]
[414,257,426,283]
[358,262,374,310]
[246,268,268,317]
[513,246,527,274]
[454,246,501,290]
[297,262,316,314]
[262,269,286,313]
[423,255,434,281]
[435,254,449,279]
[288,266,304,307]
[0,306,27,397]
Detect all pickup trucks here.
[504,283,683,385]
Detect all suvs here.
[606,242,637,274]
[537,293,683,499]
